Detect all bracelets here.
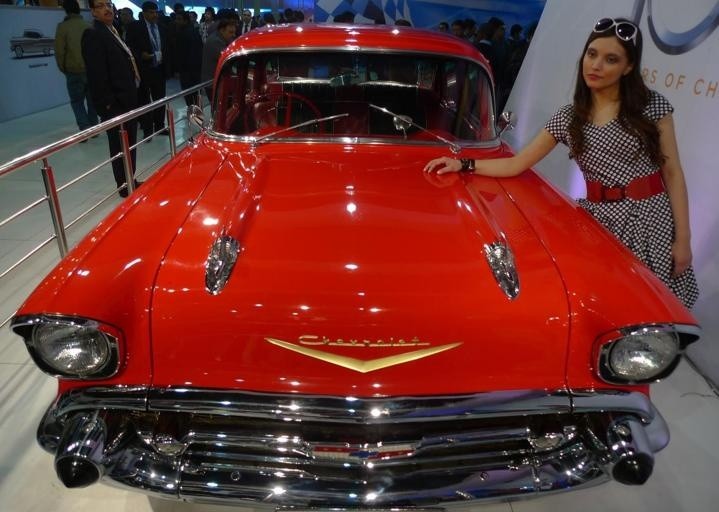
[461,159,476,176]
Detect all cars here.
[10,22,701,512]
[10,29,55,58]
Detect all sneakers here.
[143,127,169,143]
[119,181,145,199]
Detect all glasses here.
[592,15,638,48]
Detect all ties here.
[150,23,159,50]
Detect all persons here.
[334,12,543,120]
[55,0,100,142]
[129,3,172,144]
[110,3,309,121]
[81,1,148,198]
[421,17,700,309]
